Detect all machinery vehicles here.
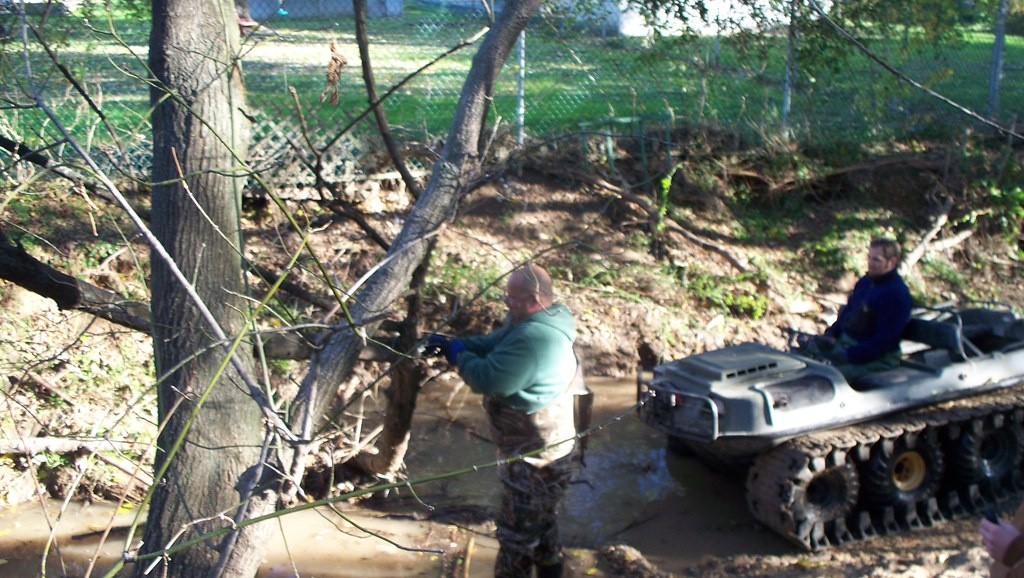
[635,296,1023,553]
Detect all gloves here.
[802,334,829,354]
[447,338,465,366]
[817,350,843,367]
[428,334,447,345]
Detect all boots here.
[535,557,564,578]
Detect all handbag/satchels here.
[570,386,594,469]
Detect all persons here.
[427,265,594,578]
[980,501,1024,578]
[798,236,913,391]
